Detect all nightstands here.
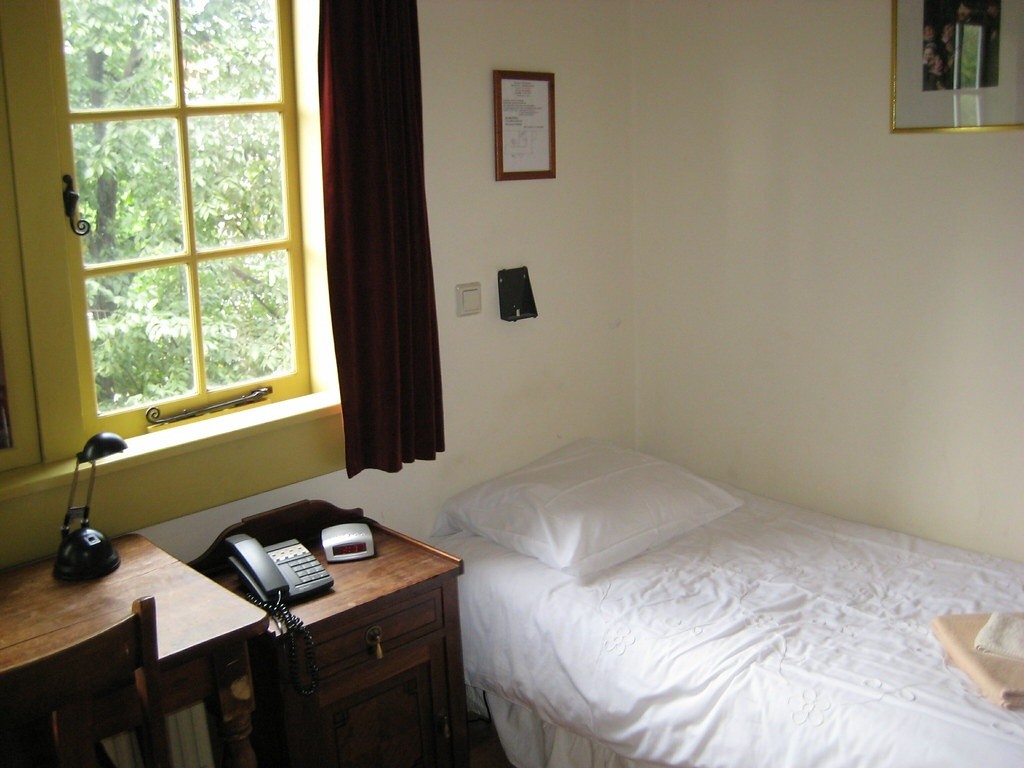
[189,498,473,768]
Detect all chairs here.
[0,596,174,768]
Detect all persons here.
[922,1,1001,91]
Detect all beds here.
[422,473,1024,768]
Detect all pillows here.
[429,439,744,579]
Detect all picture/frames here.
[493,70,557,181]
[889,1,1024,136]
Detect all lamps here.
[48,430,128,583]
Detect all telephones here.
[226,533,335,609]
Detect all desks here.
[0,533,270,768]
